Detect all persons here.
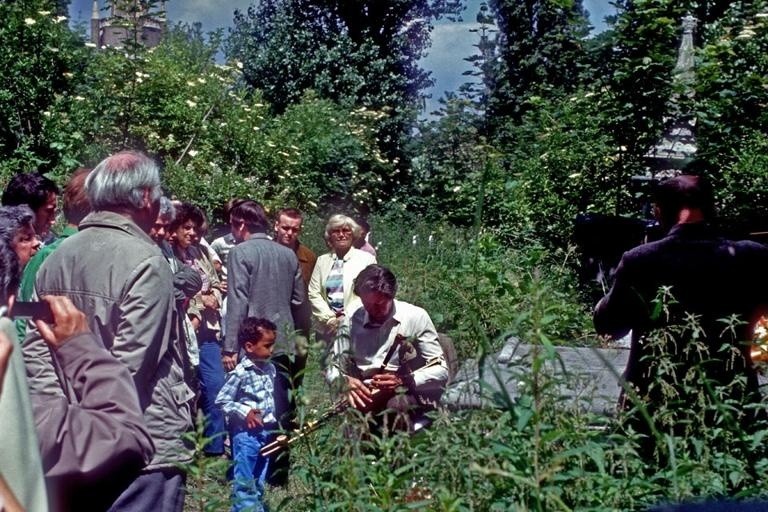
[0,232,155,509]
[0,165,379,459]
[215,315,290,512]
[326,262,449,441]
[17,148,201,509]
[592,171,767,496]
